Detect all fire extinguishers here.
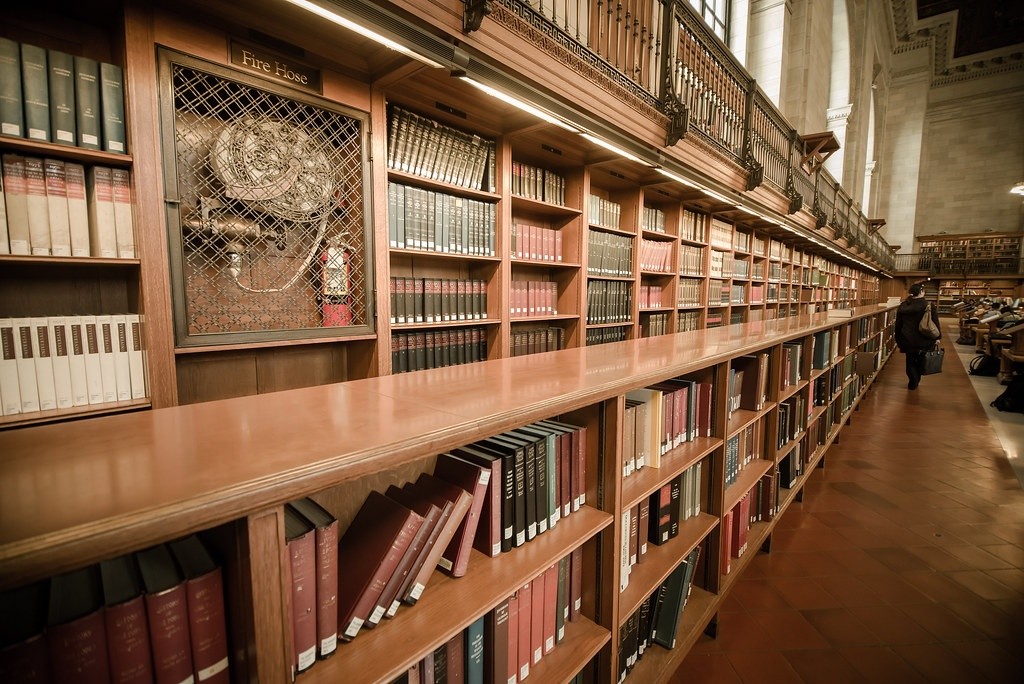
[322,232,356,327]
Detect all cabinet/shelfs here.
[0,303,899,684]
[916,231,1024,275]
[0,0,896,435]
[937,278,1024,316]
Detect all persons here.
[964,298,1020,324]
[895,283,942,390]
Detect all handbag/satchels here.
[919,304,940,336]
[921,344,945,376]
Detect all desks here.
[950,296,1024,414]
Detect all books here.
[0,1,897,684]
[919,239,1024,314]
